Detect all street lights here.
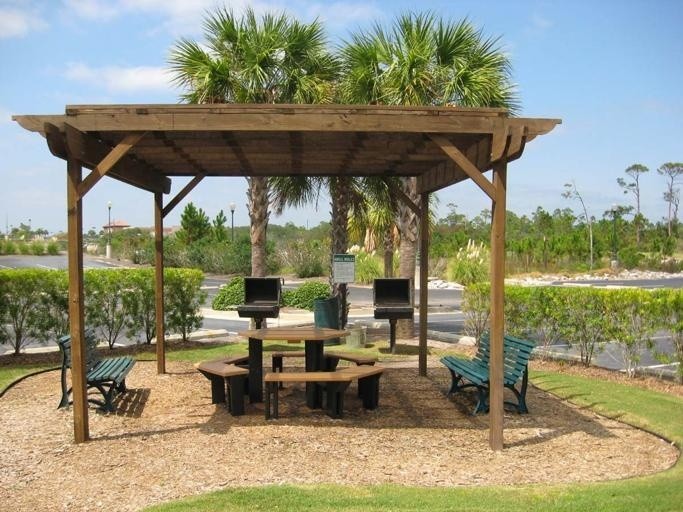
[611,202,619,271]
[229,201,236,242]
[106,201,113,259]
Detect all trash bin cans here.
[344,325,367,350]
[313,296,341,346]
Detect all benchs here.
[57,326,135,414]
[437,327,537,418]
[196,349,385,420]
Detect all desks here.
[238,324,350,409]
